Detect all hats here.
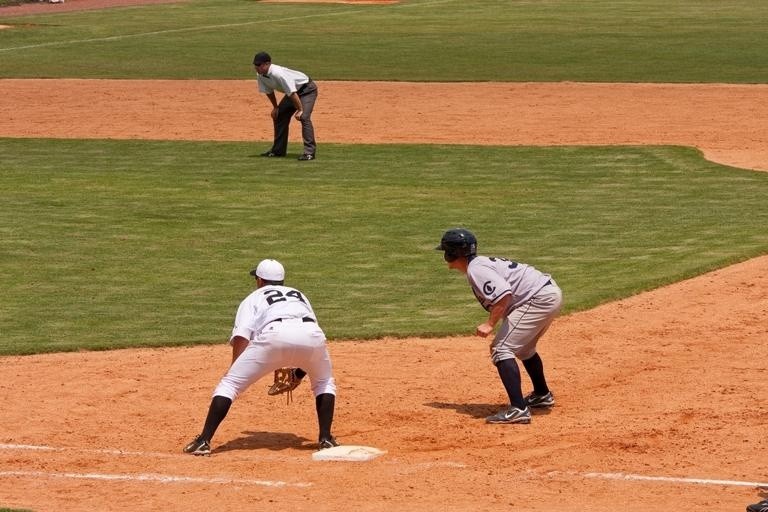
[249,259,285,282]
[253,52,271,65]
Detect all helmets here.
[434,228,477,262]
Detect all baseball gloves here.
[267,364,302,396]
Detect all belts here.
[275,316,314,323]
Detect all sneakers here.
[183,436,210,455]
[297,154,315,161]
[523,389,555,407]
[261,151,287,156]
[486,406,532,424]
[318,434,340,451]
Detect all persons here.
[432,227,564,426]
[252,51,318,161]
[181,259,343,456]
